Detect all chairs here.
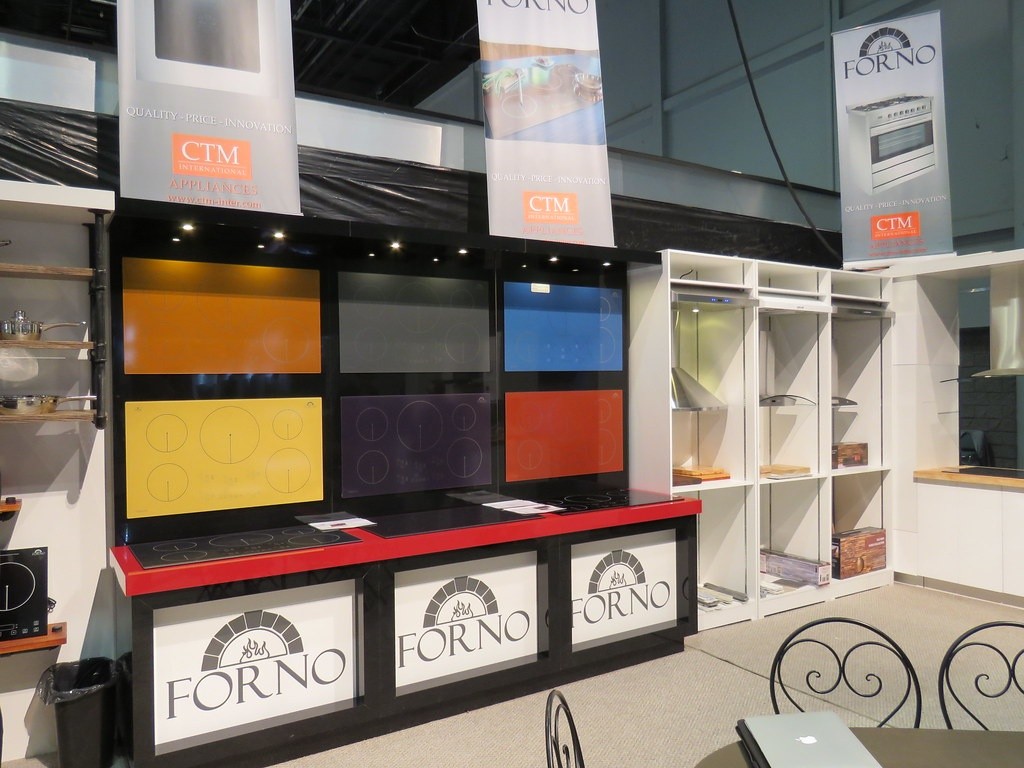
[770,616,922,729]
[938,621,1024,731]
[544,689,585,768]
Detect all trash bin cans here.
[47,657,119,768]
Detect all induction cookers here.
[0,546,49,642]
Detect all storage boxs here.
[832,441,868,469]
[760,549,831,587]
[832,526,886,581]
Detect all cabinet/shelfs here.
[0,178,115,658]
[629,248,894,633]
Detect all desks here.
[695,727,1024,768]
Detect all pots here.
[0,310,83,340]
[0,393,98,414]
